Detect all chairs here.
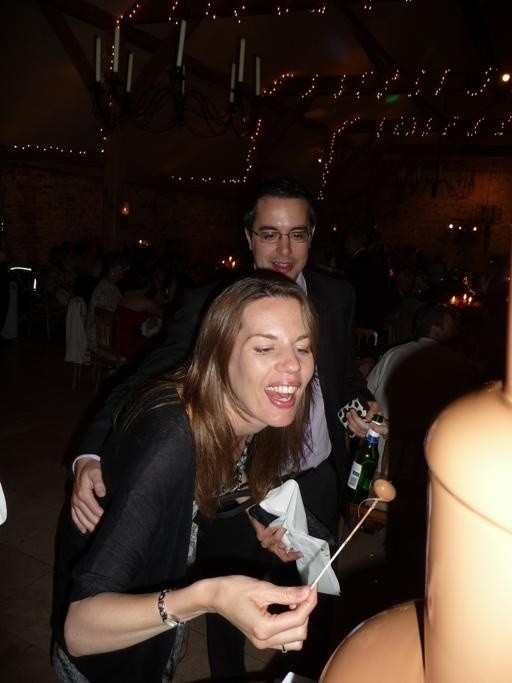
[333,434,391,580]
[27,285,418,397]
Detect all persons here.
[0,225,468,513]
[50,265,320,683]
[71,179,390,683]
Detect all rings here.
[281,644,290,655]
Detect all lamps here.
[87,0,268,138]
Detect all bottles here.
[345,414,384,508]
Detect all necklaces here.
[220,435,251,490]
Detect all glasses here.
[251,228,313,244]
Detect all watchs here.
[155,588,188,629]
